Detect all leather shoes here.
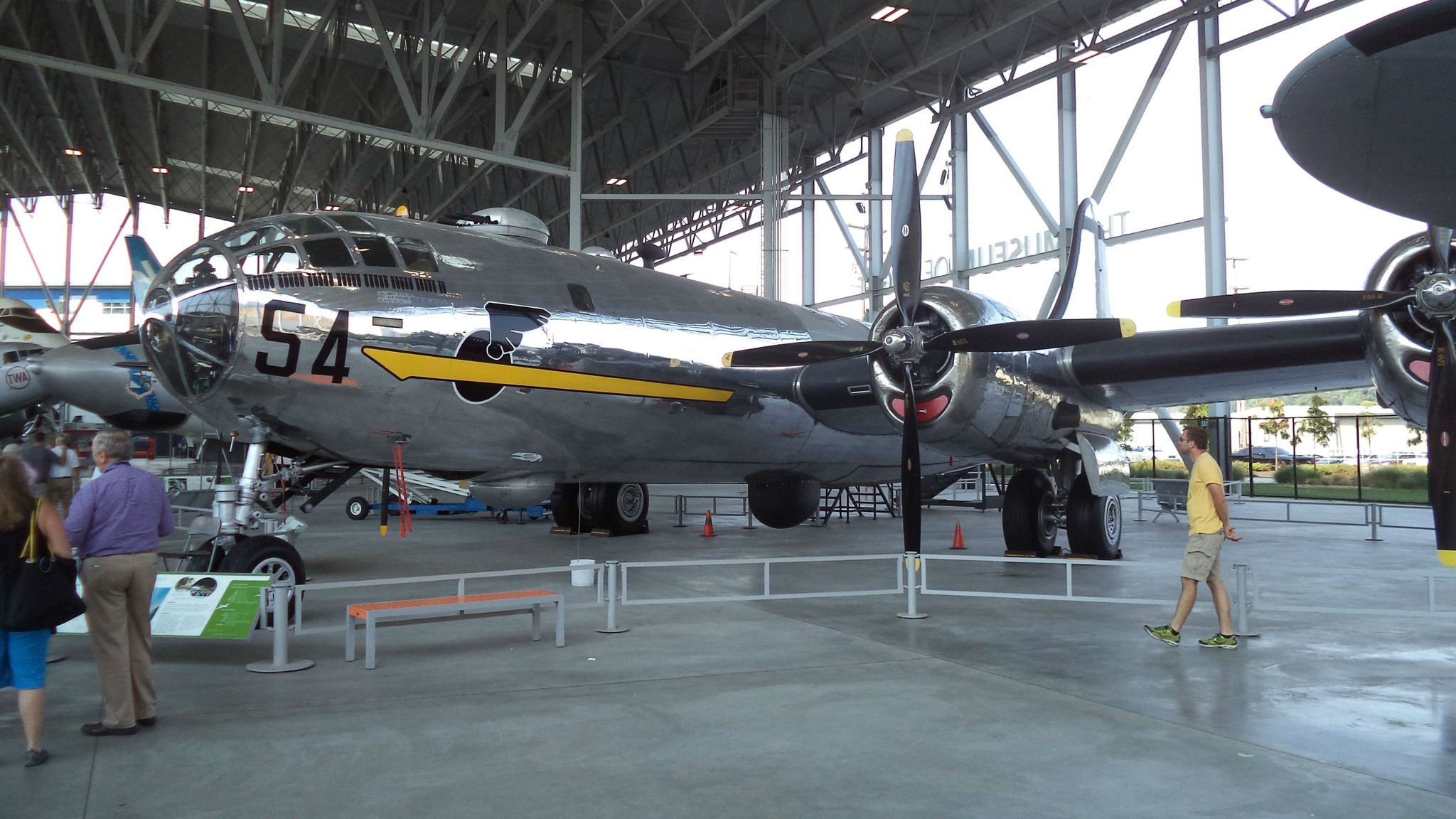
[81,722,138,736]
[136,716,156,726]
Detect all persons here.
[1144,424,1243,650]
[24,432,68,482]
[0,455,87,767]
[46,436,80,520]
[63,427,175,736]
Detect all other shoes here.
[24,750,49,767]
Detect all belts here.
[53,476,71,478]
[120,549,155,555]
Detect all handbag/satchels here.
[5,495,88,632]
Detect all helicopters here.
[3,0,1453,544]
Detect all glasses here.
[1180,438,1193,442]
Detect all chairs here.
[157,516,222,572]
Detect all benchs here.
[345,591,566,669]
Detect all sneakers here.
[1144,625,1181,647]
[1199,633,1238,650]
